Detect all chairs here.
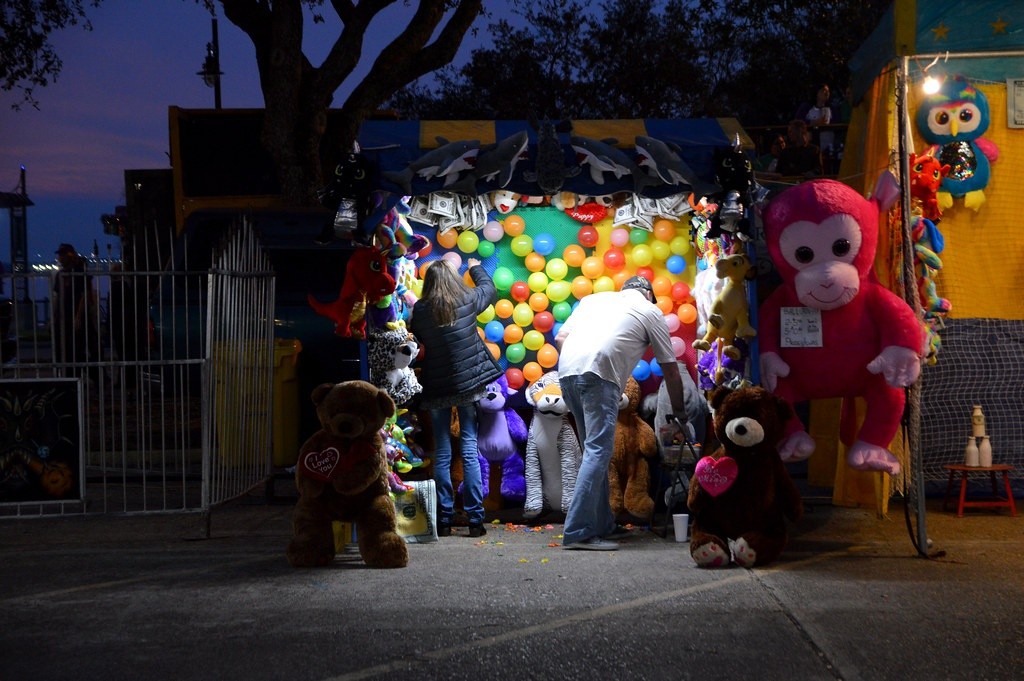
[645,415,700,540]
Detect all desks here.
[943,463,1018,518]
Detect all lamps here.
[196,43,224,88]
[922,69,939,94]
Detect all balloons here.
[410,215,698,389]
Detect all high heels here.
[469,521,486,537]
[438,521,450,535]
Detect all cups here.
[673,514,688,543]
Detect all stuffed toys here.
[638,363,711,506]
[916,77,998,214]
[382,110,721,214]
[759,178,928,474]
[446,372,528,497]
[282,380,409,567]
[686,385,804,567]
[607,374,655,518]
[910,147,951,365]
[691,146,757,391]
[308,152,427,497]
[524,370,583,521]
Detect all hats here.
[622,276,657,304]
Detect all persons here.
[553,275,690,551]
[767,119,824,178]
[52,242,155,387]
[410,257,505,534]
[795,81,854,154]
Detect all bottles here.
[964,436,979,467]
[979,435,992,468]
[971,405,986,438]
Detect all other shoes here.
[607,526,633,539]
[562,536,620,550]
